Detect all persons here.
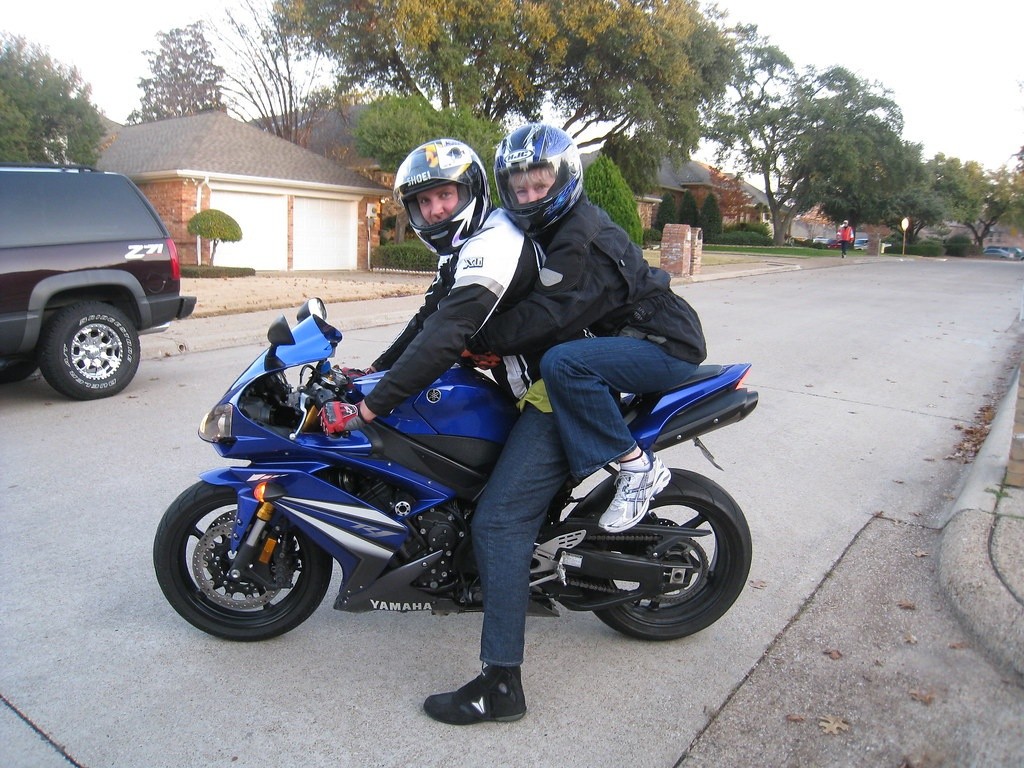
[459,125,707,532]
[317,139,619,726]
[836,220,854,258]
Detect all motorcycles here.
[151,301,760,642]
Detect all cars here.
[854,238,869,251]
[825,239,843,248]
[984,245,1024,261]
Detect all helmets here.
[494,124,583,232]
[399,139,491,255]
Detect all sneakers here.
[598,449,671,533]
[423,661,526,725]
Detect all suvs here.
[0,164,198,401]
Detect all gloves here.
[461,349,501,370]
[342,367,375,380]
[317,402,371,439]
